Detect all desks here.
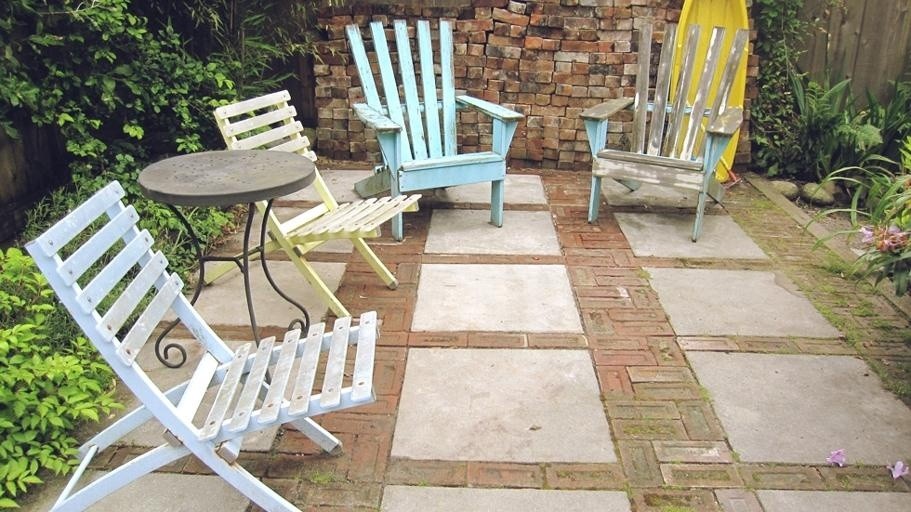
[137,149,315,385]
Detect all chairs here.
[24,178,382,512]
[346,19,524,240]
[580,21,751,244]
[197,90,422,318]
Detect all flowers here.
[802,137,911,297]
